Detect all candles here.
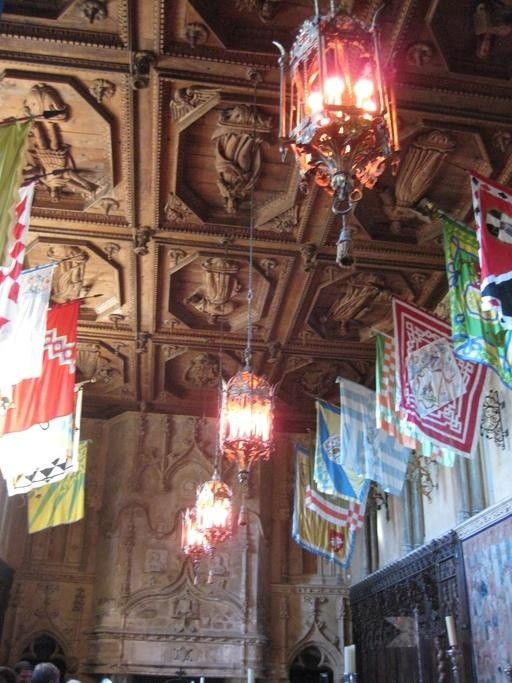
[444,615,459,647]
[248,668,255,683]
[344,644,356,674]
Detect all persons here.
[0,667,17,683]
[32,662,60,683]
[14,661,33,683]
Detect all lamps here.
[219,185,289,486]
[273,1,404,270]
[181,506,207,570]
[196,449,236,559]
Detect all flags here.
[0,168,73,328]
[439,157,512,329]
[0,256,77,387]
[437,209,512,390]
[336,376,412,498]
[0,294,104,437]
[375,328,455,468]
[1,379,96,497]
[28,439,92,535]
[0,412,74,481]
[391,293,492,460]
[312,399,372,506]
[0,110,66,266]
[305,430,370,534]
[290,443,357,570]
